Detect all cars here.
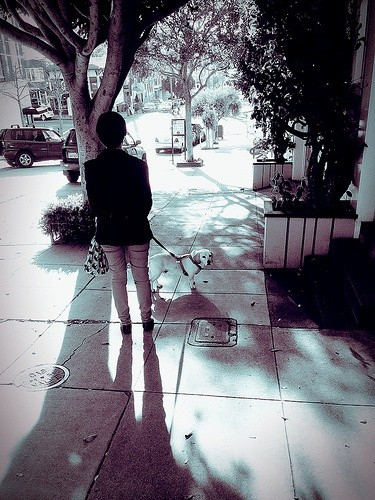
[191,123,206,145]
[141,97,186,114]
[154,125,189,155]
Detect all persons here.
[83,112,155,334]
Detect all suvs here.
[0,124,65,169]
[28,105,54,121]
[61,125,148,183]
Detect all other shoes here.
[120,322,132,334]
[143,318,154,332]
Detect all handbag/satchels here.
[83,227,110,277]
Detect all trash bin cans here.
[215,125,223,139]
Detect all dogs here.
[148,249,214,293]
